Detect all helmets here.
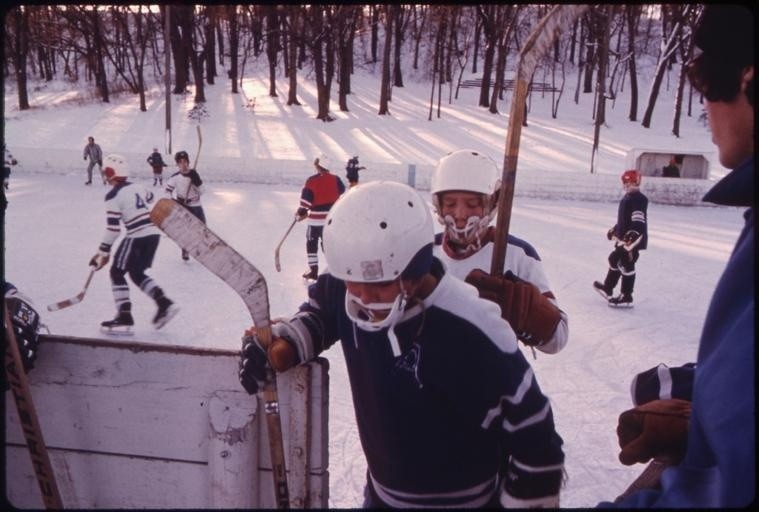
[101,154,129,183]
[322,182,436,358]
[426,148,505,246]
[620,170,642,193]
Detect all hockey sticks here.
[150,198,291,508]
[183,126,201,204]
[275,220,295,271]
[491,5,588,276]
[47,267,95,311]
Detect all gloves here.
[238,317,320,396]
[618,398,696,464]
[625,232,638,246]
[464,269,559,346]
[190,169,204,186]
[89,249,111,269]
[607,225,617,241]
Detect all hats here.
[174,151,188,162]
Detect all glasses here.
[683,52,747,101]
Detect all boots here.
[595,267,620,297]
[303,252,320,279]
[140,280,172,322]
[103,285,135,325]
[608,272,635,304]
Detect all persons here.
[4,143,20,190]
[346,155,365,188]
[4,272,41,397]
[612,3,757,511]
[84,135,205,333]
[592,170,649,307]
[293,156,345,282]
[424,151,569,356]
[663,159,680,177]
[239,179,566,511]
